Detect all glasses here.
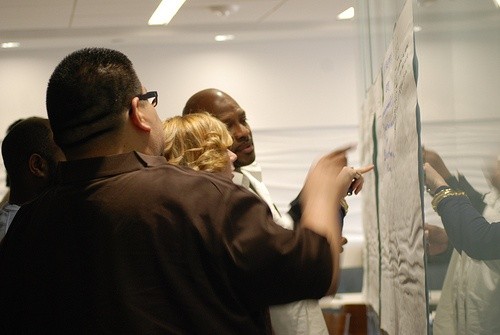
[129,91,158,115]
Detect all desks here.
[321,288,441,335]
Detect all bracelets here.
[431,188,470,214]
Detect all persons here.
[422,146,500,335]
[0,46,360,335]
[183,89,330,335]
[162,110,375,233]
[0,117,66,242]
